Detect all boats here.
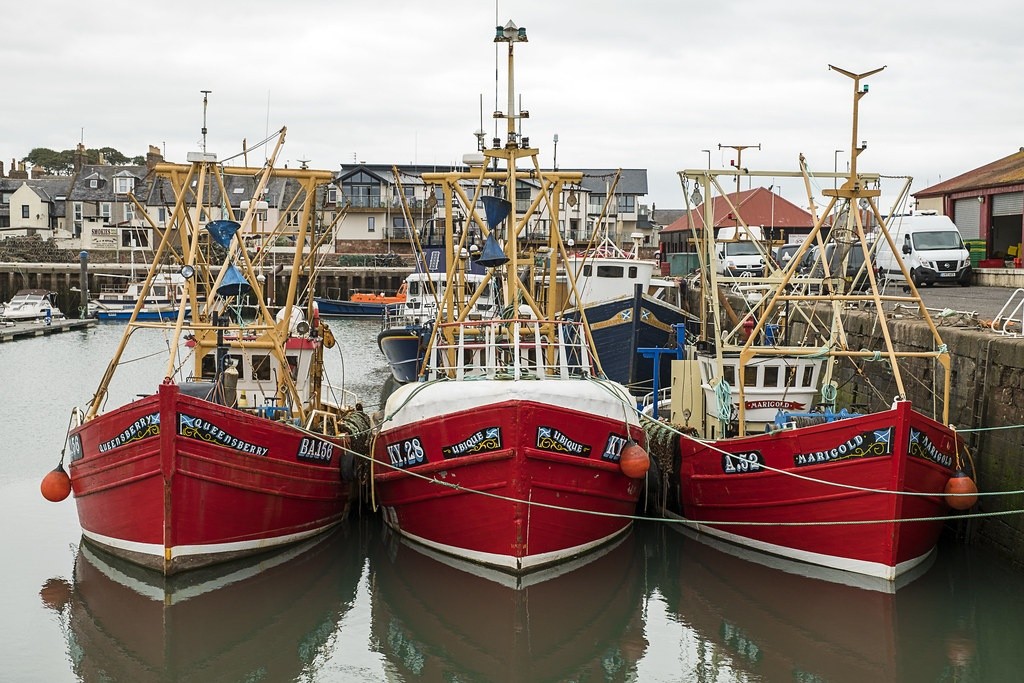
[71,91,371,576]
[654,519,1024,683]
[75,123,669,324]
[649,64,966,583]
[68,520,364,683]
[363,16,653,580]
[91,206,199,322]
[365,532,648,683]
[0,294,64,322]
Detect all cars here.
[800,241,880,294]
[774,243,815,291]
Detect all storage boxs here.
[963,239,1022,268]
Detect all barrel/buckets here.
[661,262,670,277]
[277,305,304,333]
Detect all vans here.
[874,209,974,288]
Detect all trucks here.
[714,227,769,286]
